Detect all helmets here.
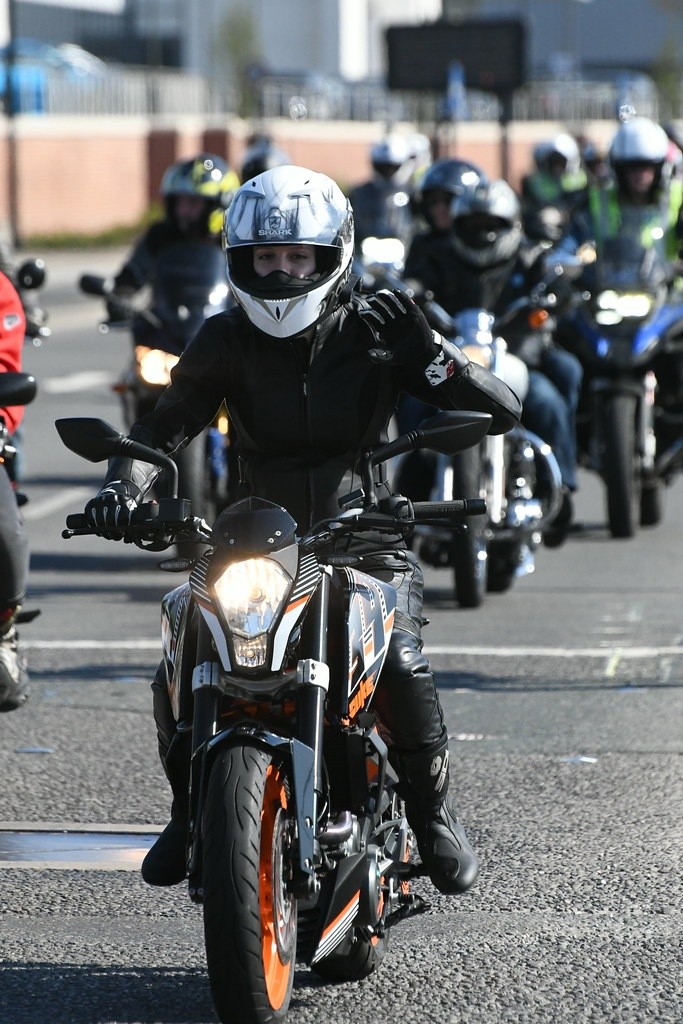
[359,117,683,275]
[240,138,288,183]
[222,164,356,338]
[164,151,239,234]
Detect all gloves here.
[85,482,145,541]
[356,287,435,376]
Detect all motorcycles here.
[0,257,51,347]
[1,368,39,713]
[334,160,595,308]
[78,267,240,564]
[49,362,491,1024]
[385,265,561,613]
[537,206,683,542]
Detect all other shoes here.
[0,614,23,648]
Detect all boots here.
[141,736,197,889]
[394,732,479,894]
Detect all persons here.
[401,159,581,495]
[570,115,683,483]
[0,266,33,714]
[87,163,524,897]
[105,150,248,510]
[423,175,577,551]
[235,118,683,294]
